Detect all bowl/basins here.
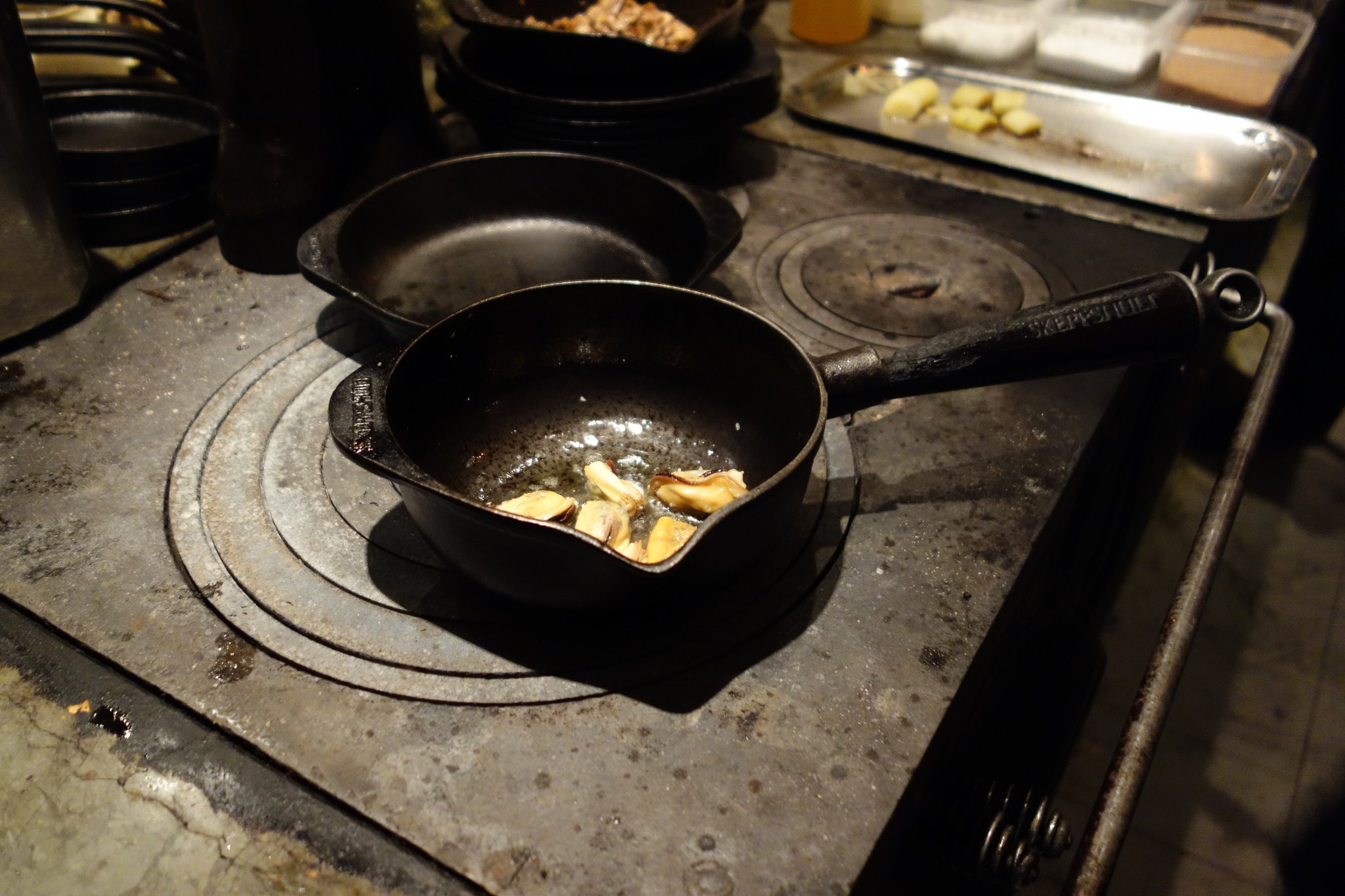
[906,0,1320,104]
[296,145,747,339]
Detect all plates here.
[20,0,788,278]
[784,47,1320,231]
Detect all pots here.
[323,261,1271,594]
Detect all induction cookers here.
[0,157,1228,896]
